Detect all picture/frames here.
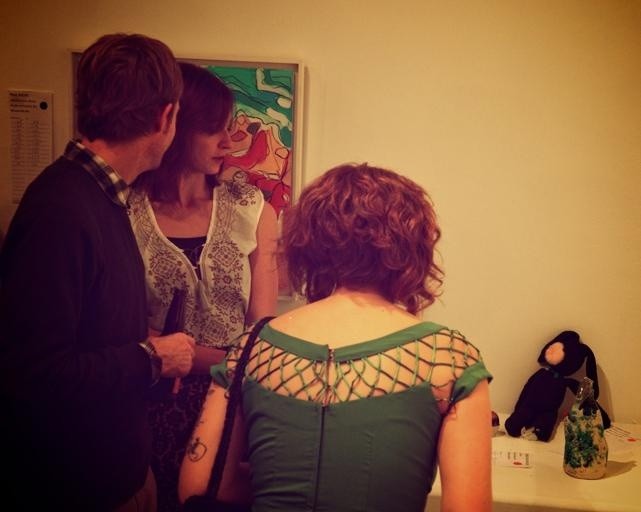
[174,58,306,302]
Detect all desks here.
[427,413,641,512]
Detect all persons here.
[171,158,499,512]
[1,31,215,512]
[112,53,289,511]
